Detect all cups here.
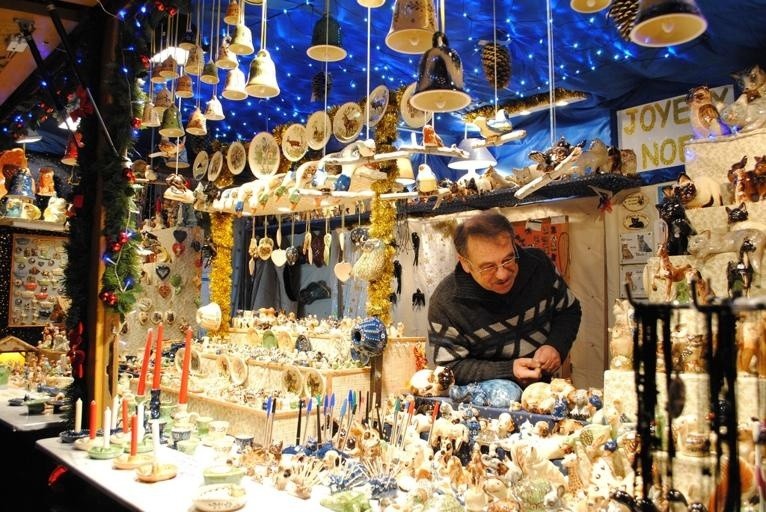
[148,416,254,462]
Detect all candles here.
[73,322,196,464]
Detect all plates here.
[247,328,311,353]
[282,366,326,403]
[174,348,248,385]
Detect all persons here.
[426,213,583,392]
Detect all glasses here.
[463,240,520,273]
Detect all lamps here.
[444,112,504,180]
[15,127,44,146]
[55,112,88,135]
